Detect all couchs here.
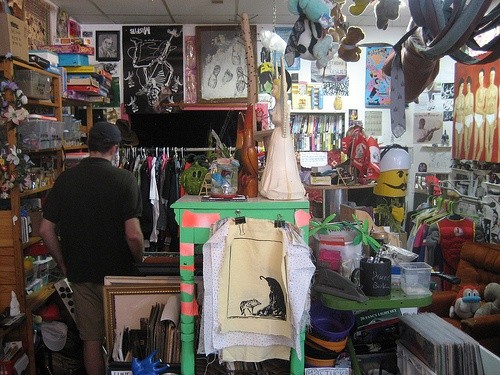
[431,242,500,358]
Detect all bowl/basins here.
[302,302,355,368]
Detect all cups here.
[351,257,391,296]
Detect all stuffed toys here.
[449,282,500,319]
[287,0,402,69]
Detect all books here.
[290,114,342,151]
[398,312,485,375]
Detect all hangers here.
[407,196,479,227]
[118,146,185,169]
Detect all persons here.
[39,122,144,375]
[453,67,500,161]
[98,35,117,58]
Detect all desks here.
[170,193,310,375]
[314,271,432,375]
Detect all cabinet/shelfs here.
[0,59,92,375]
[288,110,347,152]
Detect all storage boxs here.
[399,261,433,295]
[0,13,112,150]
[309,176,331,186]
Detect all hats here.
[87,121,121,152]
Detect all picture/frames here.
[101,275,204,375]
[96,31,121,62]
[273,27,300,70]
[195,25,259,104]
